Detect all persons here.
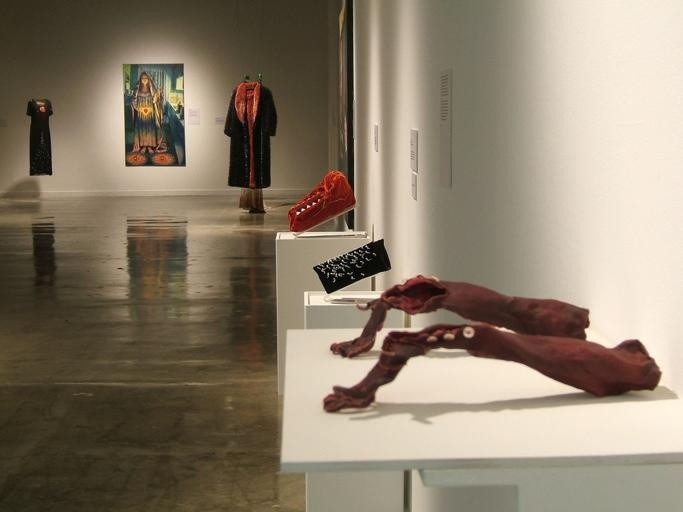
[130,71,165,155]
